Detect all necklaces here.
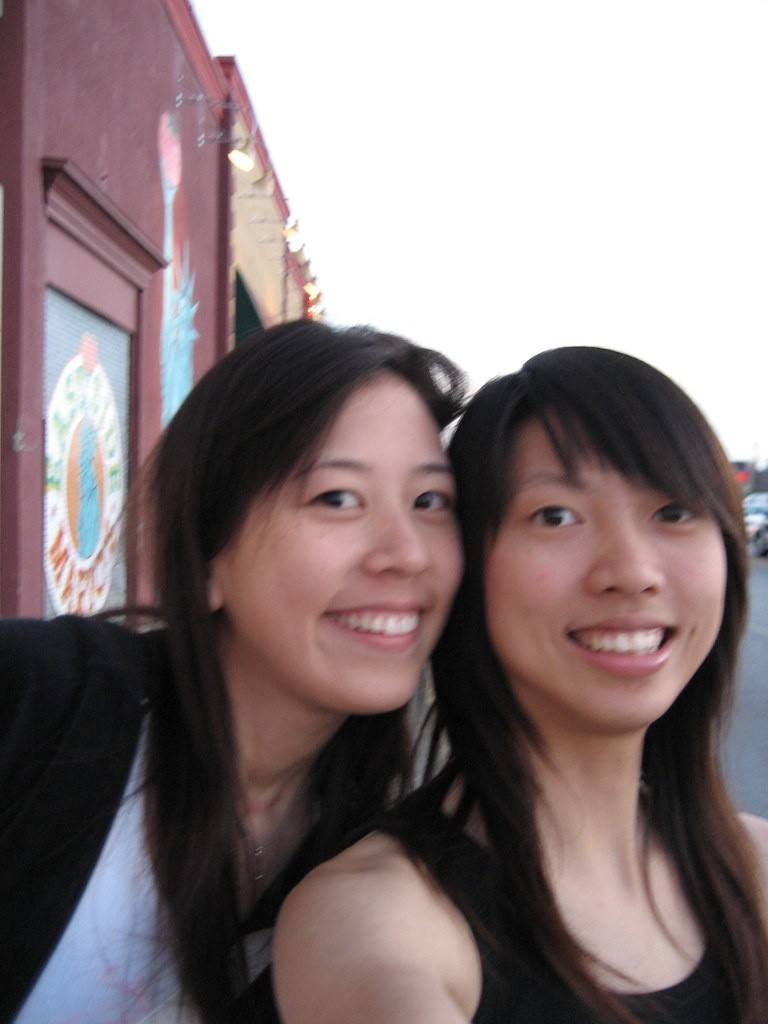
[240,767,310,883]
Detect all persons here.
[1,319,470,1024]
[272,345,767,1024]
